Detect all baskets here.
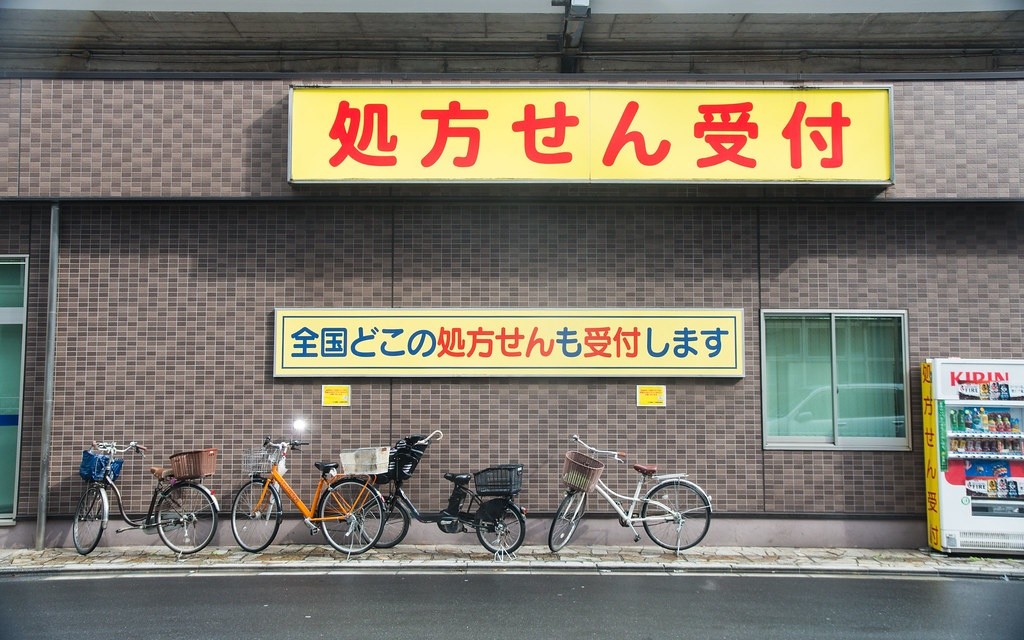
[339,446,390,475]
[169,448,216,479]
[242,448,280,476]
[562,451,605,492]
[80,451,125,482]
[368,449,397,484]
[473,464,524,495]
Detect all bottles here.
[971,408,982,432]
[958,410,965,432]
[1003,417,1011,433]
[995,418,1004,432]
[964,411,973,432]
[88,440,96,455]
[951,410,958,430]
[989,417,996,431]
[980,408,989,432]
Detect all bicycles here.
[548,434,713,558]
[72,440,221,561]
[229,434,386,560]
[359,438,528,562]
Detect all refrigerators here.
[921,358,1024,554]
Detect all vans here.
[768,383,905,438]
